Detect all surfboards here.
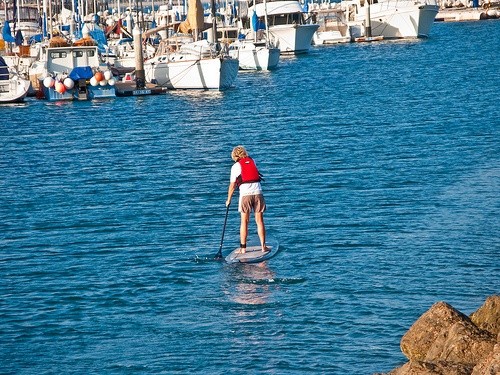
[224,233,280,264]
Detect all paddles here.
[213,199,232,261]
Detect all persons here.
[226,146,270,254]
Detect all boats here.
[224,233,280,265]
[0,0,500,107]
[246,0,320,55]
[353,0,440,39]
[142,0,240,92]
[310,8,352,45]
[0,0,31,102]
[48,0,117,102]
[229,10,281,71]
